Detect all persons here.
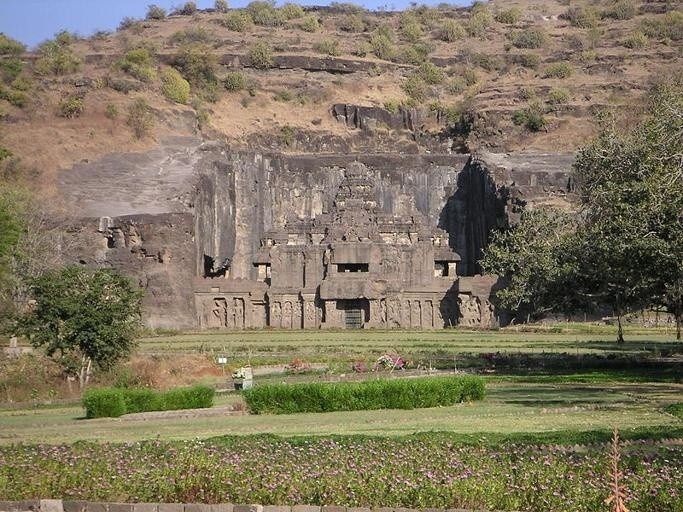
[212,299,226,328]
[232,299,244,327]
